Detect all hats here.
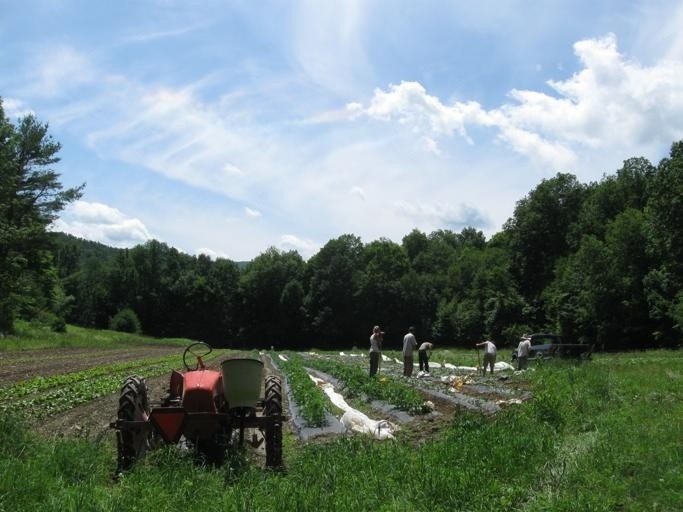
[373,326,379,331]
[521,334,528,339]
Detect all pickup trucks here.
[511,334,590,358]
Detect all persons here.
[403,326,418,376]
[476,340,497,377]
[369,325,383,377]
[517,334,531,369]
[418,342,435,373]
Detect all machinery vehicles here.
[108,339,283,466]
